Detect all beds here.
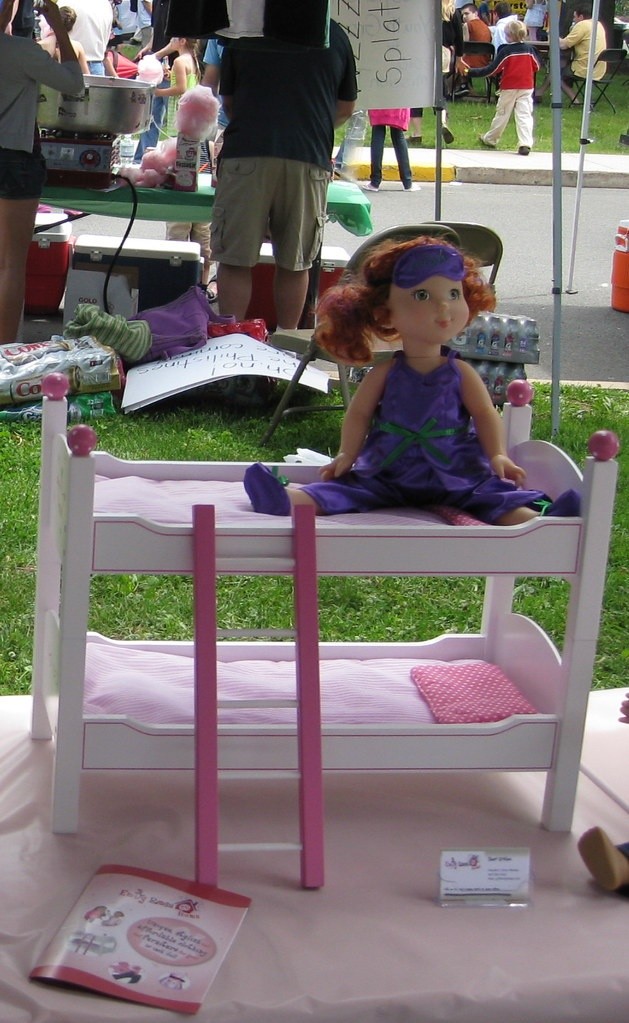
[28,373,619,884]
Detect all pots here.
[36,75,152,135]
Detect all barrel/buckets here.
[610,220,629,313]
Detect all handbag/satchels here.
[523,0,547,27]
[127,284,236,364]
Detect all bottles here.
[443,309,540,406]
[120,134,134,167]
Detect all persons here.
[243,225,582,531]
[463,19,540,155]
[1,0,609,342]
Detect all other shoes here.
[519,147,530,155]
[406,135,422,145]
[455,82,470,96]
[479,133,495,148]
[402,183,420,191]
[362,184,378,192]
[443,126,454,144]
[536,95,542,104]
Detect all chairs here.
[452,40,495,103]
[569,49,629,115]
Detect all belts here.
[87,61,101,63]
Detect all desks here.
[36,179,373,328]
[523,40,554,72]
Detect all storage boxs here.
[22,214,207,326]
[238,244,352,336]
